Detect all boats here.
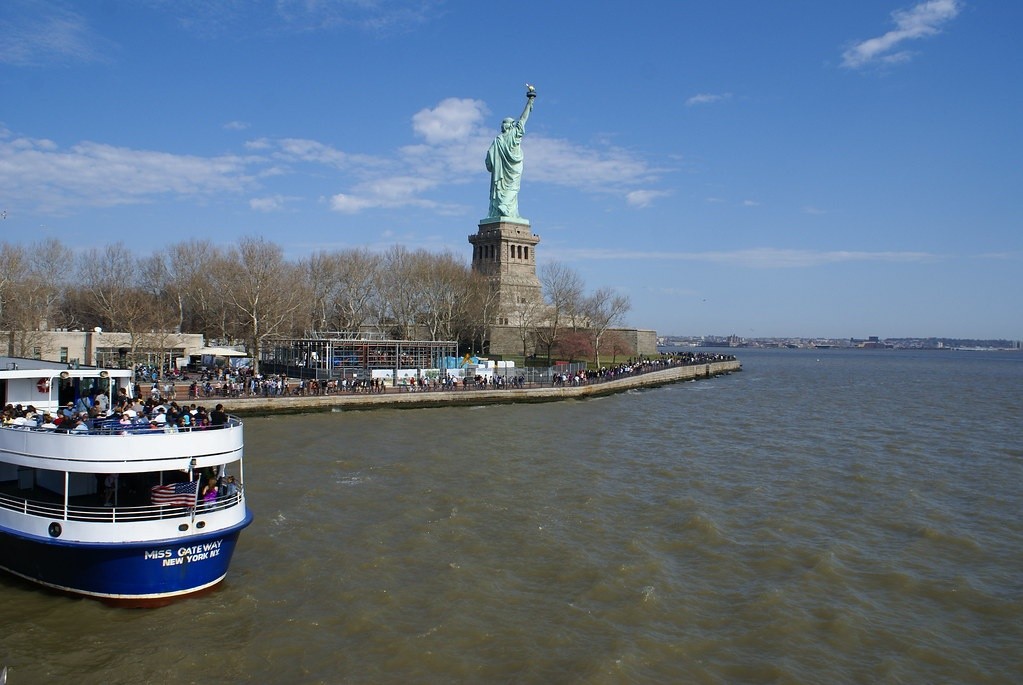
[0,356,255,608]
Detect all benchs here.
[87,412,168,434]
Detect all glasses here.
[191,420,195,421]
[182,421,185,422]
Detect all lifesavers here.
[38,378,53,393]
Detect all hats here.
[119,388,126,394]
[67,402,74,406]
[43,411,50,414]
[168,418,175,423]
[157,407,164,412]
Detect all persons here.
[298,378,386,396]
[485,96,534,217]
[107,358,188,400]
[0,389,229,435]
[553,351,736,386]
[404,375,458,392]
[462,374,525,389]
[188,364,292,399]
[104,474,119,506]
[186,464,236,509]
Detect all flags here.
[151,480,197,508]
